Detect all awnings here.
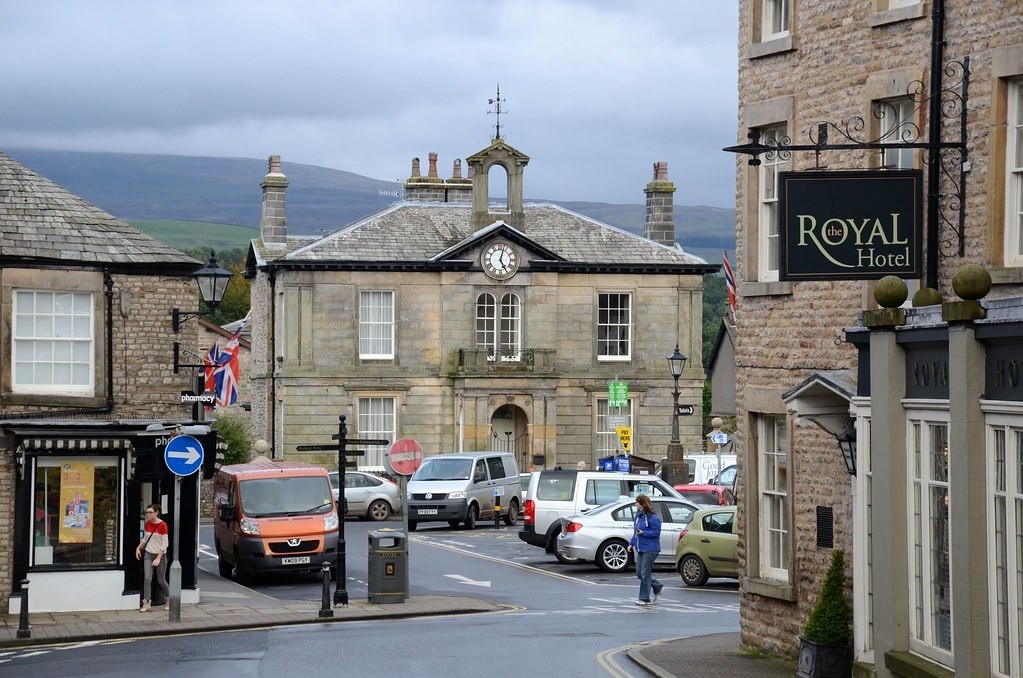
[16,438,137,486]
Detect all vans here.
[406,451,524,531]
[213,460,341,584]
[518,466,687,554]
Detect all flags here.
[722,254,736,308]
[199,319,246,410]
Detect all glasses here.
[146,511,156,514]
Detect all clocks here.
[481,240,521,281]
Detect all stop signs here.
[387,438,423,475]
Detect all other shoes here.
[635,599,652,605]
[653,587,664,602]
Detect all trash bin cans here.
[367,527,406,604]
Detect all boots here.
[164,596,170,610]
[140,599,152,612]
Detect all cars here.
[327,471,402,521]
[518,472,532,512]
[558,465,738,586]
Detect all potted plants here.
[797,548,854,678]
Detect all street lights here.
[661,344,689,485]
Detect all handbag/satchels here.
[138,548,145,561]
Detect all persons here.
[463,466,485,481]
[627,494,664,605]
[136,504,169,612]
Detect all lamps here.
[172,249,234,334]
[834,426,858,476]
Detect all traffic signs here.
[332,434,340,440]
[677,405,694,416]
[345,451,365,456]
[344,439,389,445]
[295,445,339,451]
[711,434,727,443]
